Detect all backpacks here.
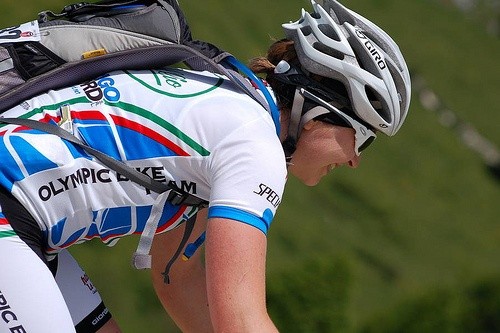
[1,0,274,210]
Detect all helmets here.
[281,0,412,137]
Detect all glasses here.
[303,87,377,156]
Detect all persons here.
[1,0,411,333]
